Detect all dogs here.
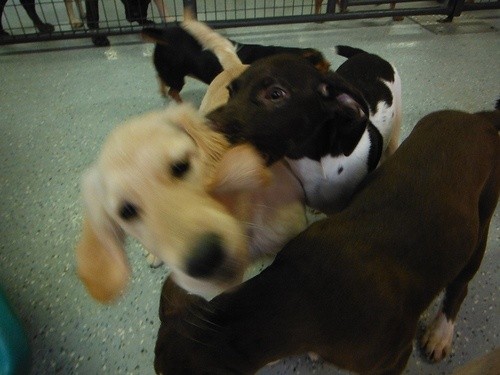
[72,15,500,375]
